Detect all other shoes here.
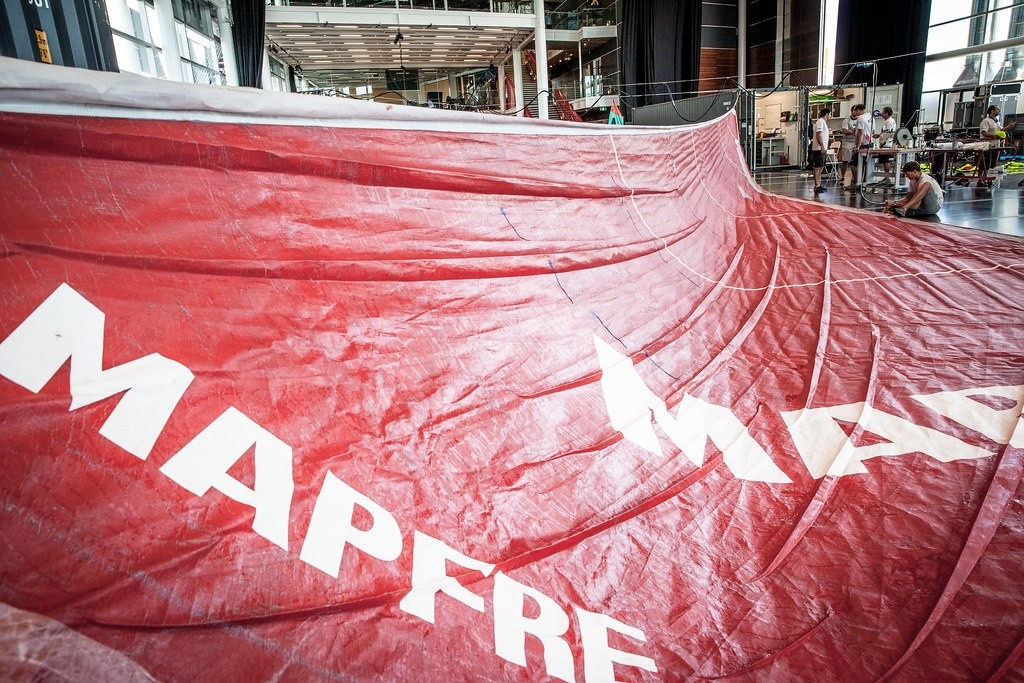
[814,184,827,191]
[976,180,988,187]
[878,179,890,183]
[893,207,905,217]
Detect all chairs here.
[822,141,844,190]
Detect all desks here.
[856,147,1013,196]
[756,138,784,166]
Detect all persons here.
[975,104,1018,187]
[871,106,897,183]
[840,103,876,188]
[879,161,943,218]
[810,108,831,194]
